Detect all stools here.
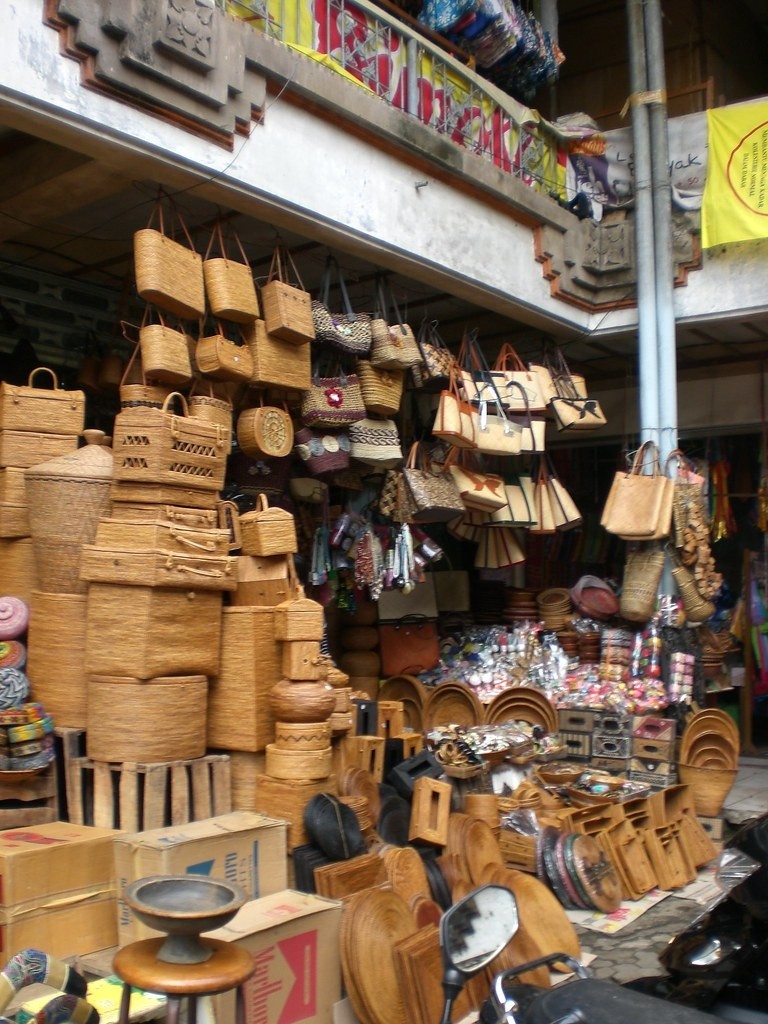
[112,936,255,1024]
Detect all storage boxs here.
[199,888,342,1024]
[82,585,223,680]
[115,814,287,951]
[336,697,454,843]
[0,820,132,971]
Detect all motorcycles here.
[440,807,768,1023]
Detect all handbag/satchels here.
[2,188,735,675]
